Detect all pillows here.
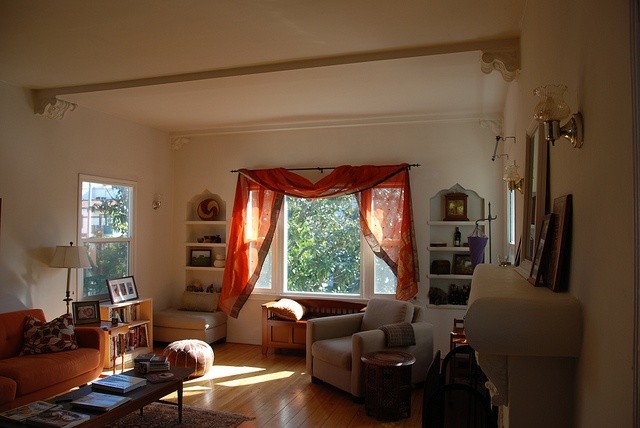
[358,297,414,330]
[178,291,219,312]
[269,299,308,322]
[19,314,80,356]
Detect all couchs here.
[154,304,228,344]
[262,298,366,360]
[0,310,105,414]
[306,298,434,404]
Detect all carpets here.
[103,402,255,428]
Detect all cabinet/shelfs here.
[183,189,226,294]
[449,318,474,380]
[427,183,485,309]
[99,297,154,370]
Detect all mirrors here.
[515,120,548,280]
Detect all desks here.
[360,351,416,420]
[99,320,130,374]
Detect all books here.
[114,304,142,323]
[111,324,149,357]
[0,400,57,422]
[143,371,181,384]
[134,354,171,374]
[26,408,91,428]
[92,374,147,394]
[69,392,132,412]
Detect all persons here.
[78,308,93,320]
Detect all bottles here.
[455,227,460,247]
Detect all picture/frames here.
[542,193,570,291]
[189,250,210,267]
[107,276,139,305]
[71,301,99,325]
[529,214,550,287]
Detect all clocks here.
[444,192,470,221]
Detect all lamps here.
[502,165,524,192]
[532,83,583,148]
[48,241,92,315]
[151,193,162,209]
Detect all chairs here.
[423,349,443,407]
[440,344,475,379]
[423,382,497,428]
